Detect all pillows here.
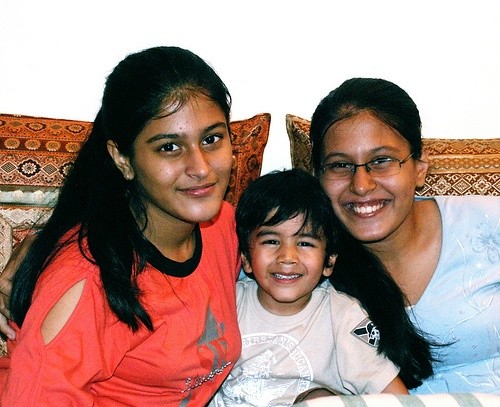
[286,111,499,196]
[1,113,272,304]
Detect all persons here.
[1,78,500,395]
[0,48,242,407]
[209,168,411,407]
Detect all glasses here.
[321,153,414,176]
